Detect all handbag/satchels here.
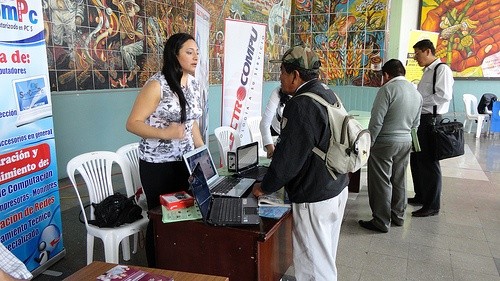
[430,117,465,160]
[477,93,500,133]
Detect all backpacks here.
[79,192,144,228]
[292,83,371,181]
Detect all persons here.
[126,33,205,267]
[251,45,350,281]
[407,39,454,218]
[259,84,294,159]
[358,59,424,232]
[0,242,33,281]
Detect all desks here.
[62,261,229,281]
[147,157,294,281]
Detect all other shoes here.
[411,206,439,217]
[392,221,401,227]
[359,219,388,233]
[408,194,422,206]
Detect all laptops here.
[183,141,269,227]
[12,75,53,127]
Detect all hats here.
[269,45,321,73]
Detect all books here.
[161,198,202,222]
[97,264,174,281]
[257,180,292,207]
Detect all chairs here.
[246,115,262,148]
[463,93,498,138]
[66,143,149,265]
[214,126,240,167]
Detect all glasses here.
[413,47,426,60]
